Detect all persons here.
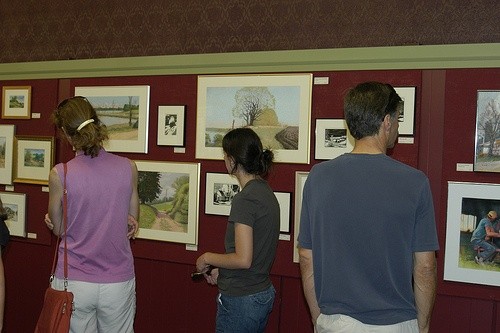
[197,130,280,333]
[298,81,439,333]
[45,97,137,333]
[0,198,10,333]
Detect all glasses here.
[191,264,215,282]
[57,96,90,121]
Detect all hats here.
[488,211,498,219]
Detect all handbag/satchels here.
[35,287,72,333]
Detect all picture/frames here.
[0,73,500,287]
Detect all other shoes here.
[476,256,483,265]
